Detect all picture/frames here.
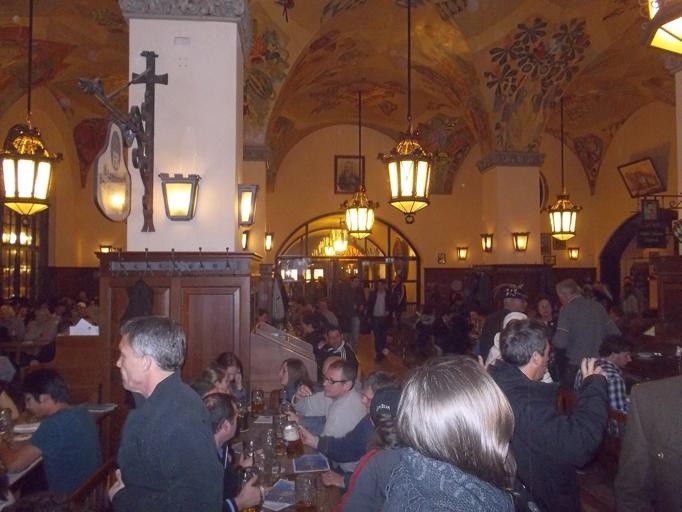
[332,154,366,195]
[641,199,660,225]
[617,156,666,199]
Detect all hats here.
[502,287,529,300]
[370,386,403,418]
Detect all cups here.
[279,420,305,459]
[294,476,317,512]
[241,467,259,512]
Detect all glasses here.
[322,375,347,384]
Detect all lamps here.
[0,1,65,227]
[99,172,128,216]
[313,230,349,257]
[264,232,273,252]
[546,96,583,241]
[455,231,581,261]
[376,1,438,224]
[99,244,112,254]
[157,172,200,222]
[237,183,258,227]
[340,89,380,241]
[241,231,249,249]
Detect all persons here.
[1,289,103,512]
[108,316,224,512]
[644,203,657,220]
[338,161,361,192]
[192,278,680,511]
